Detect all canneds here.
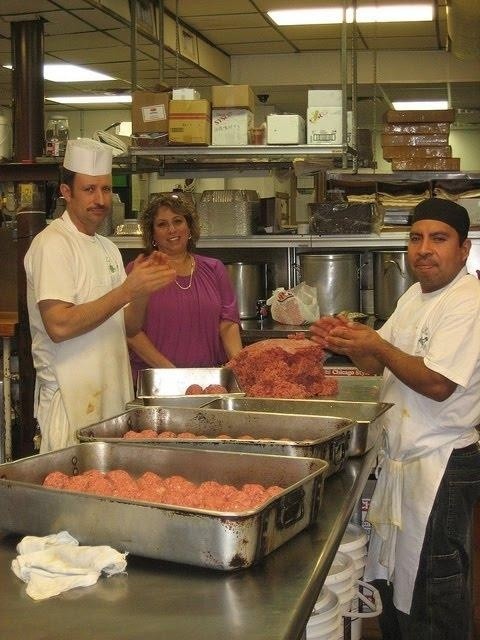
[255,300,272,330]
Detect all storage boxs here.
[130,85,347,146]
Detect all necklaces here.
[174,251,195,290]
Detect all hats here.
[63,140,112,176]
[411,198,470,247]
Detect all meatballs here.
[42,469,285,512]
[123,430,313,442]
[186,384,228,394]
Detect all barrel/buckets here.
[304,524,383,640]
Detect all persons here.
[309,197,480,639]
[124,190,244,401]
[22,167,177,455]
